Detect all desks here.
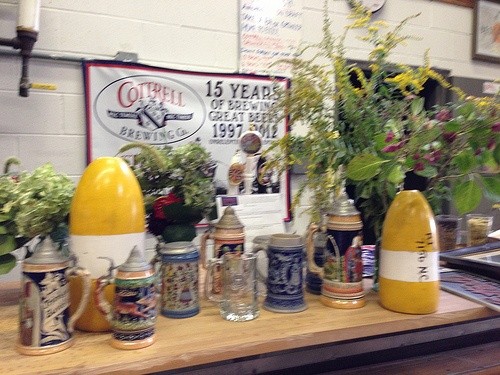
[1,295,500,375]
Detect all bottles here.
[321,195,367,309]
[377,190,440,315]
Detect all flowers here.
[117,137,217,216]
[0,159,76,272]
[248,0,499,236]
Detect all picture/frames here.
[472,1,500,64]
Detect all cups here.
[157,241,201,319]
[434,214,462,252]
[94,245,158,350]
[16,237,92,356]
[261,233,310,314]
[203,253,261,322]
[200,207,246,293]
[305,240,327,294]
[466,213,494,247]
